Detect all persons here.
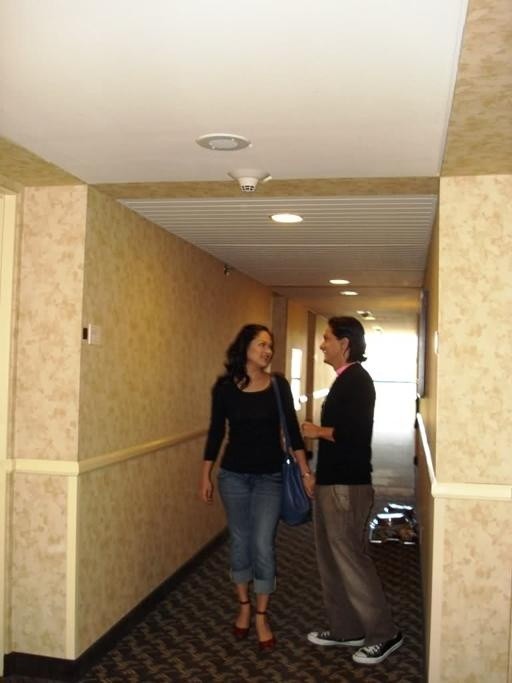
[198,321,318,653]
[301,312,407,666]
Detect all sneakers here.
[306,629,366,647]
[352,628,404,665]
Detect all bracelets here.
[301,470,313,479]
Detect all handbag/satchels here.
[280,452,311,526]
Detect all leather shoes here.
[234,599,252,641]
[255,610,276,655]
